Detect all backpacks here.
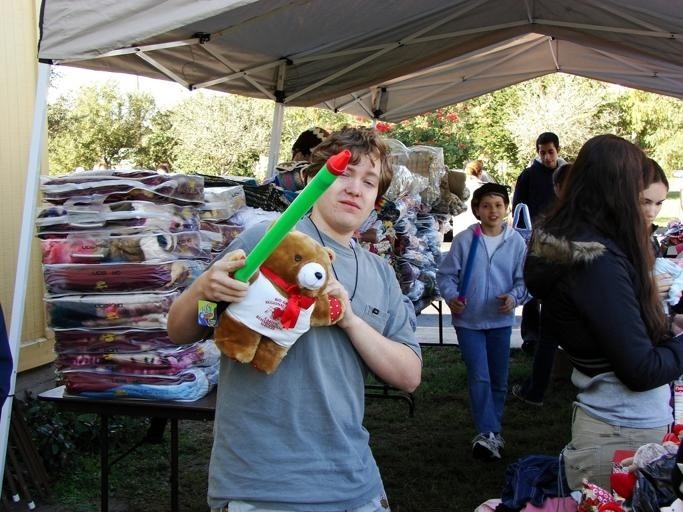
[495,453,570,511]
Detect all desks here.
[363,293,447,417]
[39,383,219,510]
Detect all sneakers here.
[472,433,505,461]
[512,385,544,406]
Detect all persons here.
[511,162,573,406]
[165,126,424,512]
[434,183,532,463]
[511,132,568,368]
[519,133,683,512]
[263,127,330,186]
[639,157,672,297]
